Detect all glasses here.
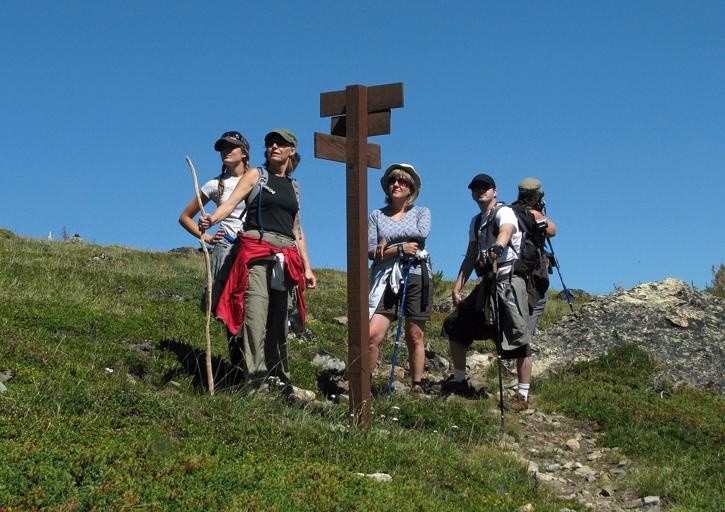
[388,177,411,187]
[221,132,247,146]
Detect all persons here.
[363,161,434,396]
[196,127,324,416]
[430,172,534,412]
[177,129,258,370]
[503,175,557,335]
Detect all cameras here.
[535,201,545,211]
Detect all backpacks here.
[488,204,541,277]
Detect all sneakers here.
[430,374,469,395]
[503,392,530,412]
[410,385,425,398]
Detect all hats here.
[215,130,250,152]
[468,174,495,189]
[381,163,422,194]
[264,128,297,147]
[518,178,543,193]
